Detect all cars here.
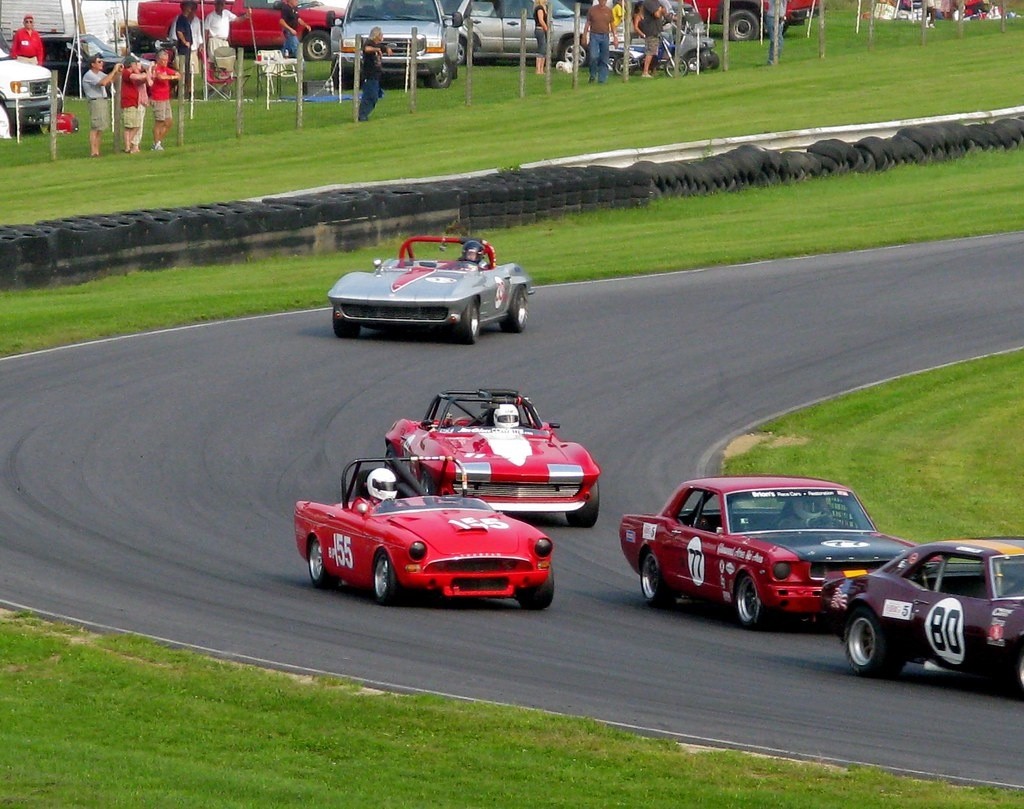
[330,0,462,92]
[440,0,593,68]
[39,32,126,96]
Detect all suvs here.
[0,29,64,135]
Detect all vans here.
[683,0,827,40]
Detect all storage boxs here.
[874,2,896,20]
[302,81,330,97]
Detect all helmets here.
[462,240,484,263]
[793,496,823,519]
[493,404,519,427]
[367,468,398,500]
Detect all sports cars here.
[326,236,537,346]
[618,476,919,632]
[383,389,602,528]
[819,535,1024,702]
[293,454,553,612]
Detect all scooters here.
[608,0,721,79]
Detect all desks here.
[255,58,298,109]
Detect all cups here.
[256,55,262,61]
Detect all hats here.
[24,16,32,20]
[123,56,140,68]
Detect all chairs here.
[257,49,297,95]
[196,44,251,101]
[362,5,375,15]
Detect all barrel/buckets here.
[47,112,78,132]
[979,12,986,19]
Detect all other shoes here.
[589,76,595,83]
[150,141,164,150]
[927,24,936,28]
[642,73,653,78]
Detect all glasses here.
[25,21,33,23]
[98,61,102,63]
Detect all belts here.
[91,98,107,100]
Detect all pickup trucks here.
[137,0,346,62]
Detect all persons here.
[926,0,936,28]
[169,1,204,101]
[358,27,392,121]
[148,50,182,150]
[581,0,618,84]
[778,496,834,528]
[81,57,124,157]
[632,0,666,79]
[533,0,549,75]
[199,0,253,61]
[121,57,150,153]
[610,0,629,28]
[366,468,398,504]
[455,240,488,270]
[279,0,311,71]
[10,15,44,65]
[765,0,787,65]
[493,404,519,427]
